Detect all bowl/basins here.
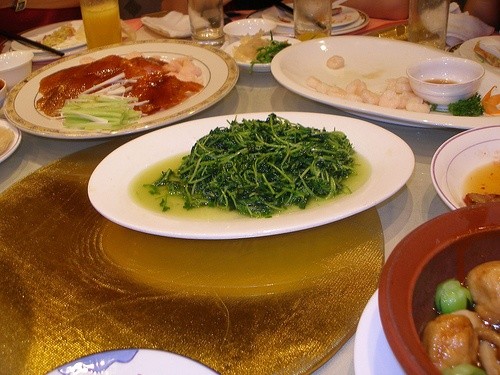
[0,79,7,108]
[222,18,277,41]
[404,55,486,113]
[377,200,500,375]
[0,50,35,87]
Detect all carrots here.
[480,86,500,115]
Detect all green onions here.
[60,73,150,133]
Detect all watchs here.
[14,0,27,12]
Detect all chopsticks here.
[271,0,327,28]
[0,29,65,56]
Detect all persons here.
[0,0,500,47]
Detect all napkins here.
[421,1,495,53]
[141,10,191,38]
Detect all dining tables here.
[0,17,500,375]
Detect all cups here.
[186,0,224,47]
[292,0,332,42]
[79,0,122,49]
[407,0,449,51]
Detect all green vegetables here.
[143,114,360,219]
[249,30,292,72]
[431,92,485,117]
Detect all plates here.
[224,35,301,73]
[246,3,371,38]
[44,348,219,375]
[429,123,500,211]
[3,39,240,140]
[353,289,406,375]
[86,110,416,241]
[269,36,500,129]
[0,118,22,164]
[9,19,87,63]
[261,3,361,29]
